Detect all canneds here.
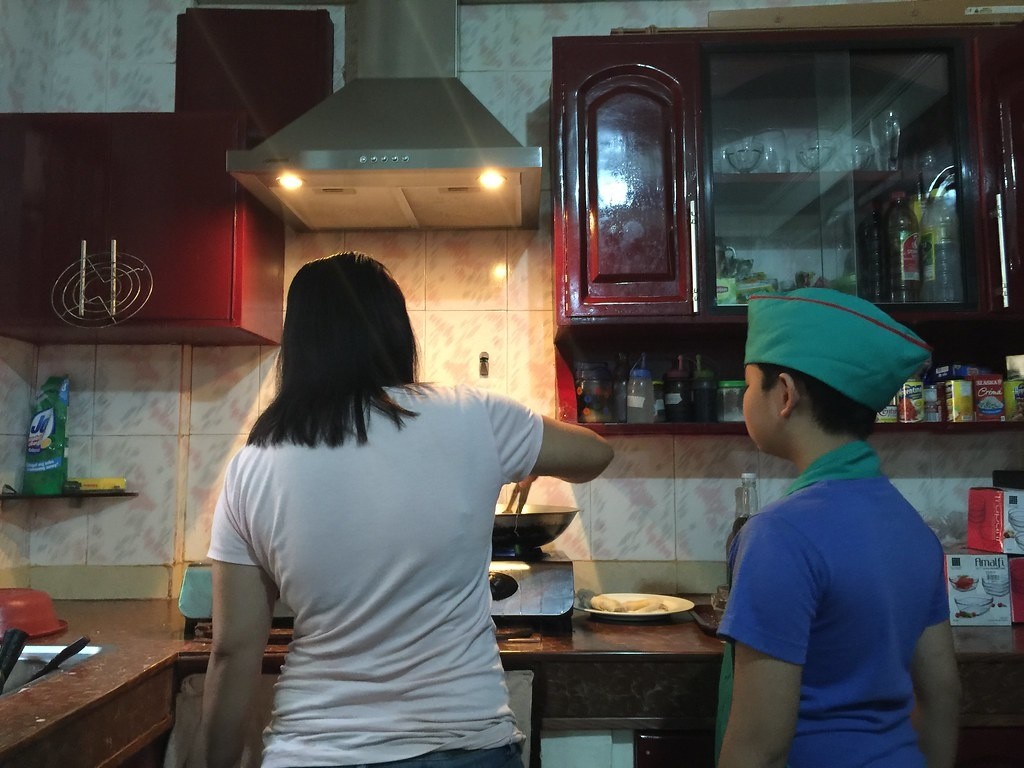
[874,379,1024,422]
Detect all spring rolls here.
[577,588,667,613]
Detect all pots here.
[492,502,580,545]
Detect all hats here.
[743,287,932,413]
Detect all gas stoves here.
[179,543,576,640]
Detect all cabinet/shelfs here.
[547,21,1023,439]
[0,111,288,347]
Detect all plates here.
[575,592,695,615]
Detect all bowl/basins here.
[981,575,1010,597]
[948,575,980,592]
[1008,507,1024,532]
[1014,531,1024,551]
[0,587,68,637]
[954,592,993,617]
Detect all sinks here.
[1,617,102,708]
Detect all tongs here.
[506,478,534,514]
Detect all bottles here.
[576,348,751,422]
[727,472,762,552]
[856,187,962,303]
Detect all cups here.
[710,585,730,611]
[714,106,902,171]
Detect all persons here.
[182,252,613,768]
[716,287,963,768]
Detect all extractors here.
[225,1,544,231]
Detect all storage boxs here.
[942,485,1023,626]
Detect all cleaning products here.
[20,373,72,493]
